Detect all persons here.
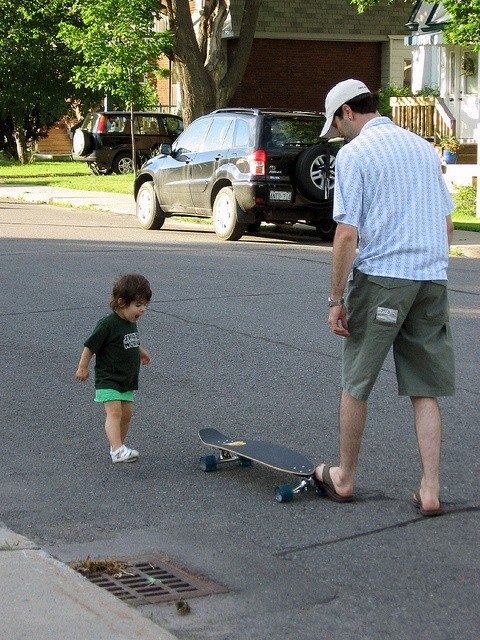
[315,77,454,516]
[76,272,153,463]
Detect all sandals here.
[110,445,140,463]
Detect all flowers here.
[440,135,464,152]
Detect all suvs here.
[134,108,348,242]
[71,111,183,175]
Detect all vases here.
[443,146,458,164]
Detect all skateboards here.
[197,427,329,503]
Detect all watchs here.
[325,296,347,307]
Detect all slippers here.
[314,463,355,503]
[411,488,444,516]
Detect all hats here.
[319,78,371,138]
